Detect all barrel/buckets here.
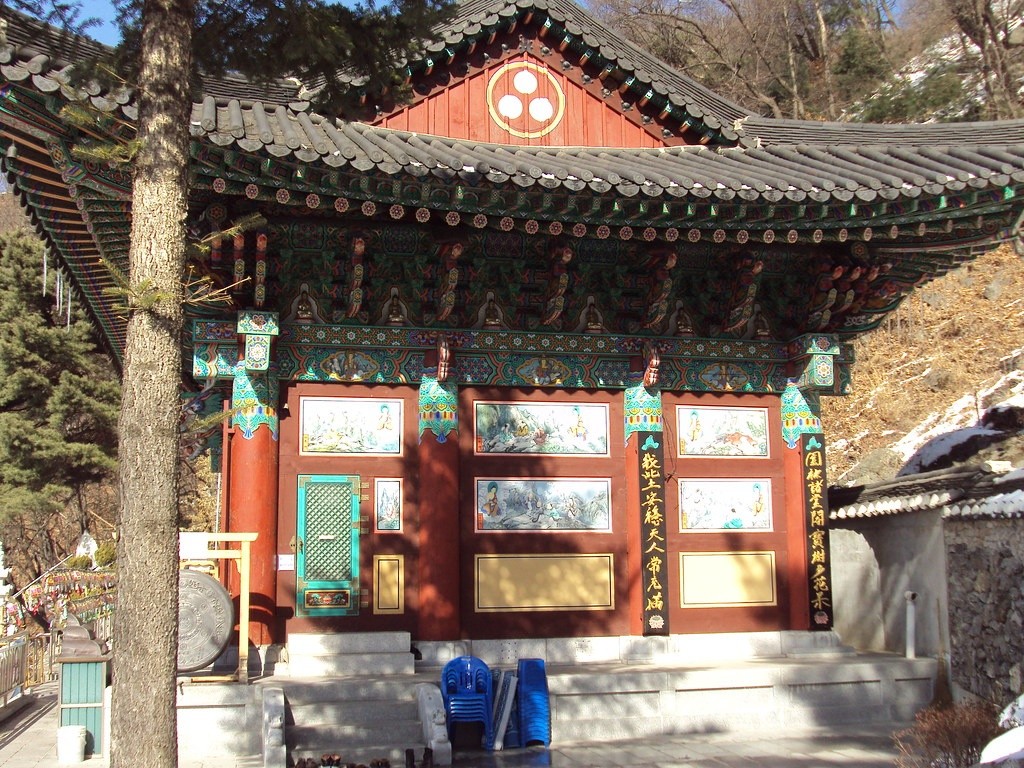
[56,725,88,765]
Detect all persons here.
[387,295,405,319]
[753,311,771,333]
[676,308,692,329]
[296,291,313,314]
[484,299,501,323]
[585,303,602,326]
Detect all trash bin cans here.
[54,612,114,759]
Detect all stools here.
[518,657,558,746]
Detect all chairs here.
[441,657,495,750]
